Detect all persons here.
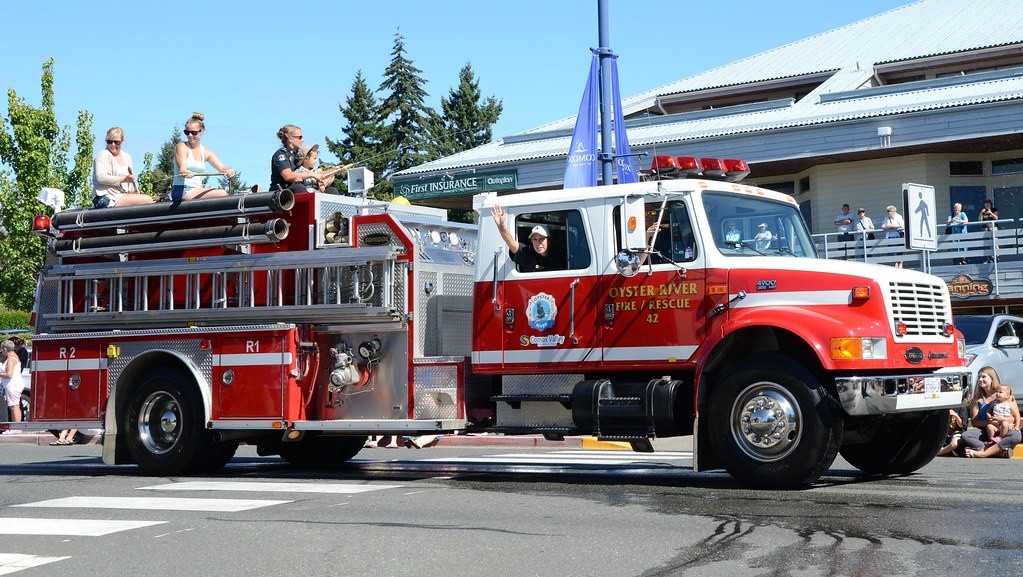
[947,203,968,264]
[726,223,741,242]
[92,127,153,208]
[171,112,235,200]
[979,200,999,263]
[854,208,874,258]
[490,204,568,273]
[985,384,1020,446]
[269,125,346,195]
[755,223,772,249]
[645,214,672,263]
[834,204,855,259]
[0,337,28,434]
[908,376,967,455]
[364,434,443,449]
[49,429,78,445]
[961,367,1022,457]
[881,206,904,267]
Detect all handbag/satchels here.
[945,226,952,235]
[899,231,904,238]
[868,233,875,240]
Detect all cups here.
[0,362,2,373]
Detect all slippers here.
[964,448,983,458]
[1003,448,1014,459]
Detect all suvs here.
[952,313,1023,417]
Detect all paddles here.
[128,165,140,194]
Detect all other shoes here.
[992,435,1004,444]
[365,441,378,448]
[985,441,995,447]
[404,441,412,448]
[386,444,399,449]
[1,429,23,435]
[407,438,423,449]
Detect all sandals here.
[49,438,75,445]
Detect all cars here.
[1,346,33,422]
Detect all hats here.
[758,223,769,228]
[858,208,865,215]
[982,199,991,203]
[529,225,550,240]
[296,142,319,166]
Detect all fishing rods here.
[322,129,474,180]
[153,172,227,183]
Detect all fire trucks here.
[0,154,973,490]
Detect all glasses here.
[183,129,203,136]
[645,210,657,216]
[291,135,303,140]
[106,139,122,146]
[886,210,894,212]
[730,224,737,227]
